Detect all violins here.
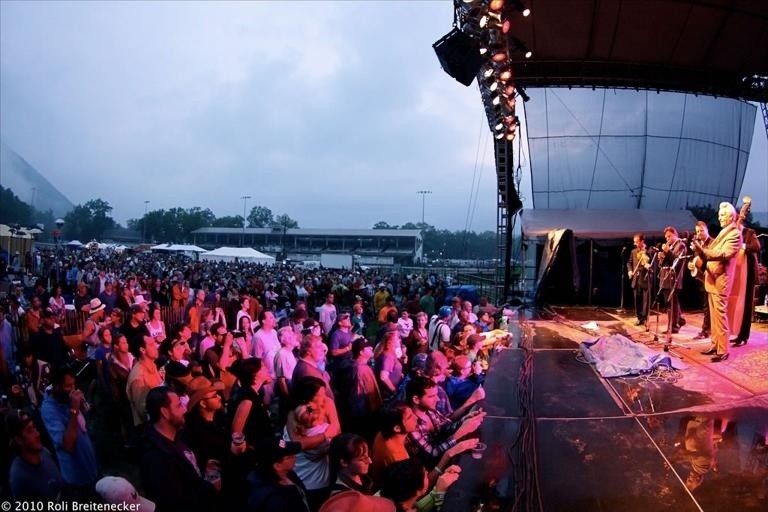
[632,249,654,273]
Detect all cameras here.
[228,331,246,339]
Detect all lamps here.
[433,0,532,142]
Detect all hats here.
[89,297,105,313]
[466,334,485,345]
[128,305,145,314]
[76,282,88,290]
[132,295,146,306]
[437,306,450,319]
[38,310,56,321]
[7,409,30,445]
[351,338,370,359]
[94,476,155,512]
[412,353,427,370]
[104,281,111,286]
[386,295,395,302]
[318,489,395,512]
[159,332,180,355]
[186,376,225,410]
[261,441,300,464]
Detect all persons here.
[669,416,715,497]
[657,226,688,335]
[689,220,715,340]
[0,247,512,512]
[730,224,762,347]
[626,233,657,326]
[689,201,743,363]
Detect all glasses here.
[199,393,217,400]
[339,316,348,321]
[303,400,313,416]
[215,334,226,337]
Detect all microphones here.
[621,244,628,255]
[681,228,690,240]
[652,244,660,254]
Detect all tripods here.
[643,249,675,345]
[645,245,696,359]
[626,254,665,338]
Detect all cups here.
[473,443,486,459]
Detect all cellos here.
[726,196,752,335]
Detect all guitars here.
[691,235,707,282]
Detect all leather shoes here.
[693,332,708,340]
[729,337,739,342]
[661,328,678,334]
[732,339,746,346]
[700,348,716,354]
[679,318,685,329]
[636,320,643,325]
[710,353,728,361]
